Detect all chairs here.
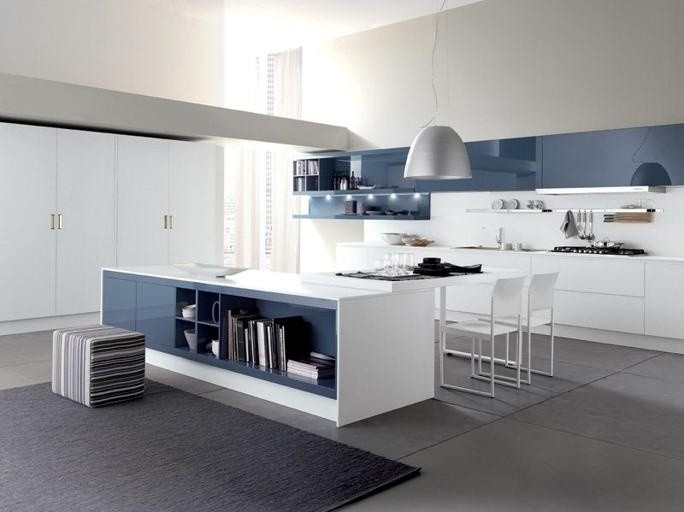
[479,272,559,384]
[440,276,526,398]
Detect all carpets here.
[0,377,421,510]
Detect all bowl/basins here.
[382,232,404,245]
[182,304,196,319]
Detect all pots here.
[589,236,623,248]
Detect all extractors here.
[534,185,668,195]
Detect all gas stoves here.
[554,245,644,256]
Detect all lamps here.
[629,125,674,186]
[403,0,473,180]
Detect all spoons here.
[579,211,586,239]
[587,210,594,239]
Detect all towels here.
[560,211,579,238]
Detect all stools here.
[51,323,146,409]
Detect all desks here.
[101,264,435,429]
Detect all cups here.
[395,252,406,276]
[384,252,395,276]
[405,253,415,273]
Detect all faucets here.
[496,228,502,246]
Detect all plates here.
[505,199,517,208]
[365,210,384,215]
[490,199,502,210]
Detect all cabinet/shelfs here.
[293,147,431,221]
[0,121,117,336]
[118,134,225,262]
[531,254,684,355]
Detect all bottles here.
[332,170,337,190]
[350,170,356,189]
[342,170,349,190]
[338,171,343,190]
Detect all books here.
[295,160,305,175]
[307,159,318,175]
[295,177,305,192]
[227,308,336,381]
